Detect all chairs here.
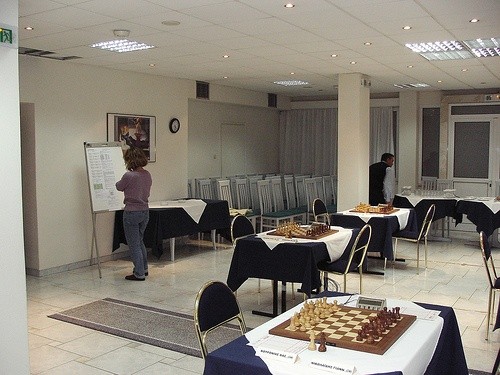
[193,279,247,358]
[480,230,500,340]
[188,173,337,305]
[391,203,435,274]
[422,176,453,238]
[304,223,372,301]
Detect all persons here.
[116,147,153,282]
[369,152,396,207]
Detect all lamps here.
[113,29,129,37]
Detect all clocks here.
[169,118,180,133]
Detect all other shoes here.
[125,271,148,281]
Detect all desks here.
[112,198,231,261]
[200,290,471,375]
[337,205,419,274]
[227,225,368,318]
[393,193,500,249]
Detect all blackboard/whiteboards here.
[84,141,128,215]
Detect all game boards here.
[269,302,417,356]
[267,226,340,240]
[349,207,400,215]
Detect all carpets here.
[47,296,255,358]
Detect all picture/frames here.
[106,113,157,163]
